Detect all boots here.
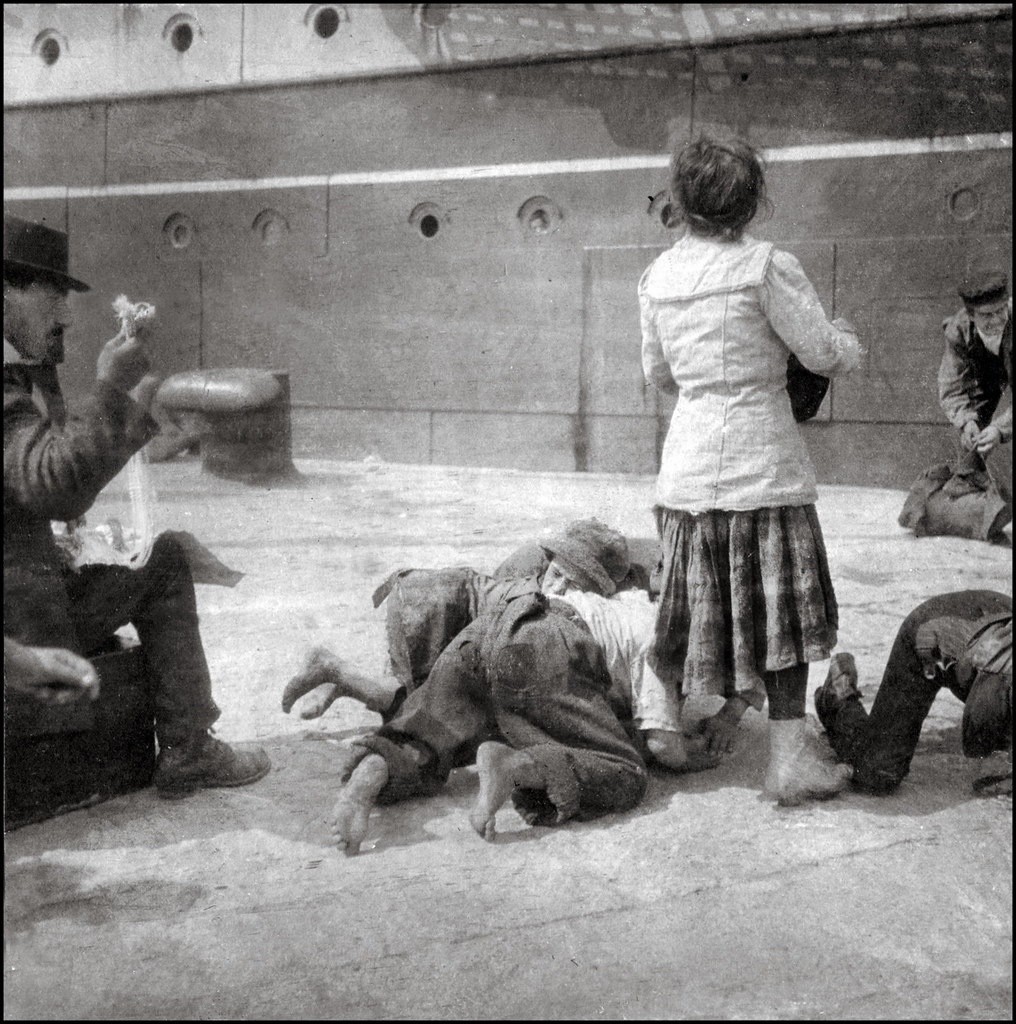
[766,717,854,808]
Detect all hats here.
[3,215,89,292]
[542,517,631,597]
[957,267,1010,313]
[787,351,829,423]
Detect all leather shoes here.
[154,740,272,799]
[815,652,864,730]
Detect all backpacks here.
[896,446,1011,544]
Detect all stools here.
[5,633,159,828]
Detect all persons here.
[4,215,271,798]
[284,517,750,852]
[937,268,1011,479]
[637,136,863,805]
[813,589,1012,797]
